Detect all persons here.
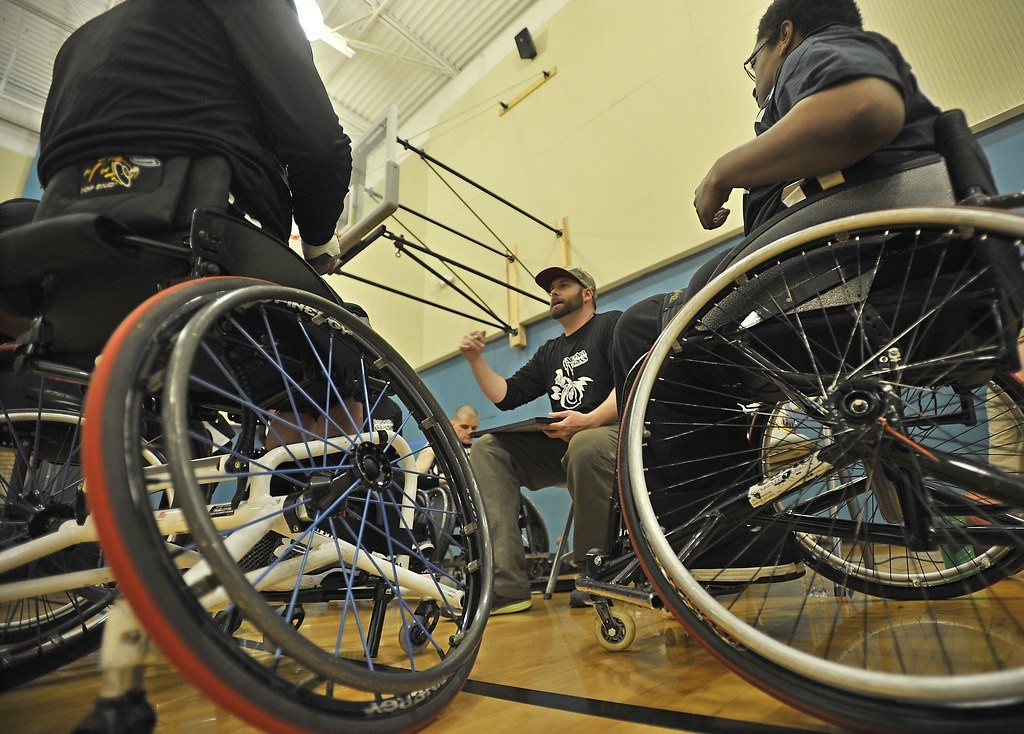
[37,0,435,591]
[459,268,651,616]
[415,404,479,523]
[611,0,942,585]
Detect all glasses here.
[744,38,771,81]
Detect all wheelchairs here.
[1,154,494,734]
[210,395,635,656]
[573,110,1023,734]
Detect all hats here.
[535,267,596,300]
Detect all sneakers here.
[570,589,614,608]
[670,523,806,586]
[236,530,435,593]
[447,592,533,617]
